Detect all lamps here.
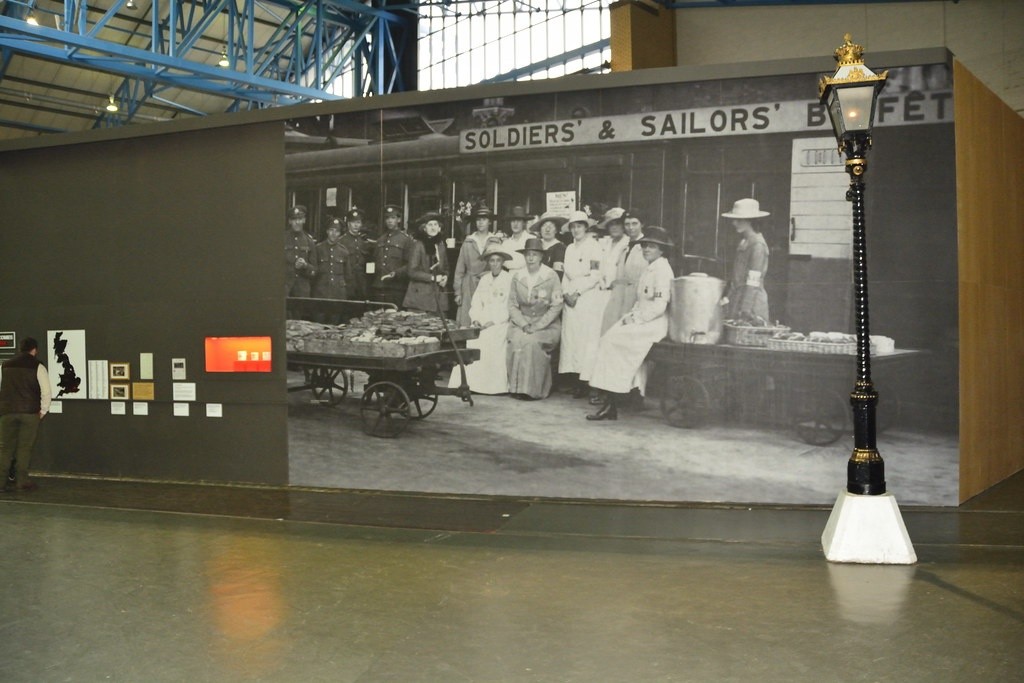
[819,30,916,564]
[106,54,119,112]
[219,3,230,67]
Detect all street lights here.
[815,33,916,564]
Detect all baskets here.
[723,322,790,345]
[766,336,896,355]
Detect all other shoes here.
[573,384,591,398]
[16,482,35,492]
[0,486,11,493]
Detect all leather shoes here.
[590,393,607,403]
[586,400,617,420]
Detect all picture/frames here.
[109,383,130,400]
[110,362,130,379]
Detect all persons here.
[286,198,771,422]
[0,337,51,494]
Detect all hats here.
[464,205,498,220]
[529,212,567,231]
[596,207,626,229]
[622,208,639,221]
[502,206,536,221]
[287,204,308,218]
[324,217,344,230]
[481,245,513,260]
[515,238,552,254]
[346,209,366,221]
[561,211,596,231]
[382,204,402,217]
[722,197,770,218]
[423,212,443,226]
[634,226,675,246]
